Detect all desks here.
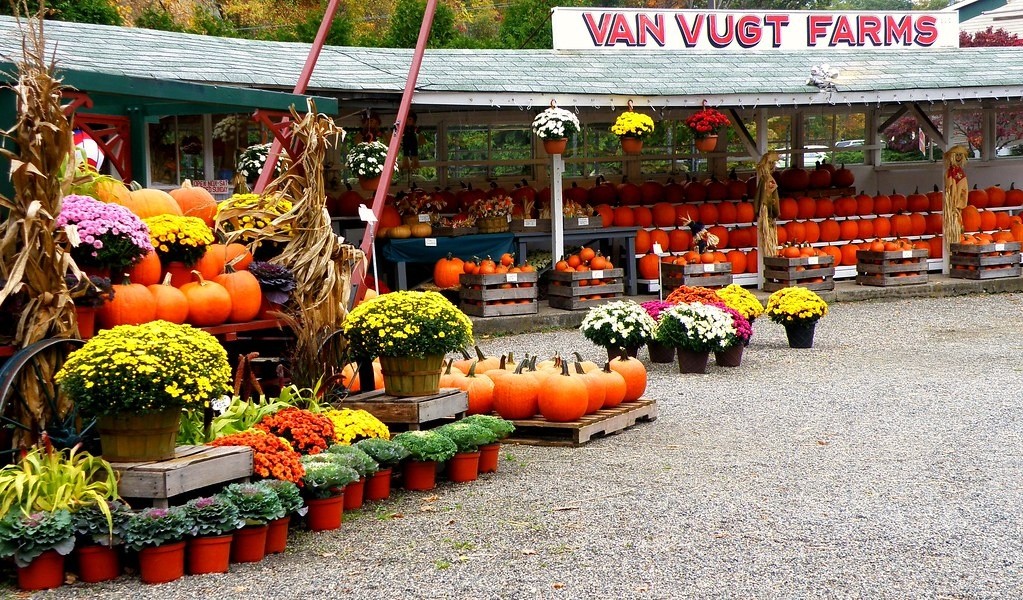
[369,231,519,291]
[513,225,642,296]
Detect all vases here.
[377,352,446,396]
[714,341,743,367]
[253,178,278,193]
[544,138,568,154]
[606,348,638,361]
[258,292,282,320]
[620,137,644,154]
[695,134,719,152]
[98,404,182,462]
[69,307,99,340]
[647,340,676,363]
[676,346,710,374]
[780,320,818,349]
[358,175,380,192]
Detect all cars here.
[967,147,1014,158]
[778,146,830,167]
[830,140,866,154]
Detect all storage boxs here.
[854,249,930,287]
[511,218,550,233]
[948,242,1022,280]
[563,216,604,230]
[432,226,479,236]
[657,262,734,300]
[762,256,835,293]
[546,268,624,311]
[457,273,540,317]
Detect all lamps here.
[810,64,839,80]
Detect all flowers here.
[211,115,245,145]
[579,285,829,354]
[468,195,515,218]
[394,190,447,217]
[683,108,731,143]
[236,142,289,180]
[531,105,581,143]
[0,193,475,487]
[610,111,655,141]
[344,139,400,182]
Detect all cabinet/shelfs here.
[635,205,1023,295]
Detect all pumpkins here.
[342,345,647,423]
[327,159,1023,307]
[81,176,261,329]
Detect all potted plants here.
[219,482,287,562]
[350,436,409,500]
[392,429,458,492]
[433,421,498,482]
[323,443,381,511]
[454,412,517,475]
[301,461,360,532]
[0,503,76,591]
[180,494,246,575]
[253,479,309,555]
[70,499,134,583]
[122,505,198,585]
